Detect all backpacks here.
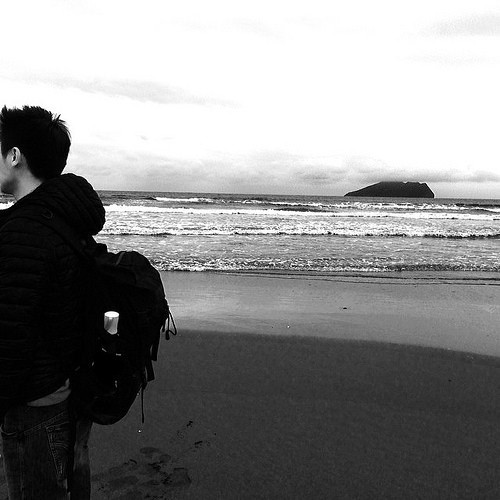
[11,207,171,426]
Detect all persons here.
[0,105,108,500]
